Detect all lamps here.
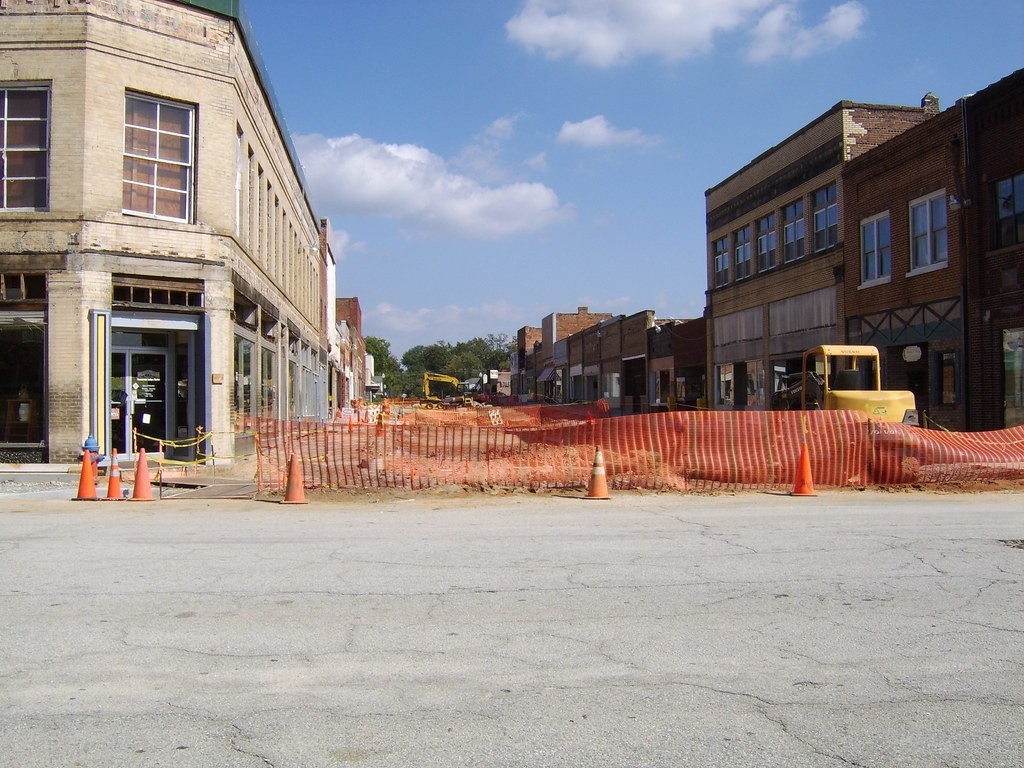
[596,329,607,337]
[350,344,358,351]
[298,241,319,256]
[655,324,662,333]
[949,190,972,211]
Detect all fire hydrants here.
[77,434,105,485]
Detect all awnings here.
[371,376,380,387]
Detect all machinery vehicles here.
[769,344,921,445]
[417,371,474,409]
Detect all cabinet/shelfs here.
[4,399,38,443]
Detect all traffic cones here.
[71,448,100,502]
[278,454,310,504]
[127,447,156,502]
[788,446,821,497]
[579,445,612,499]
[97,447,129,501]
